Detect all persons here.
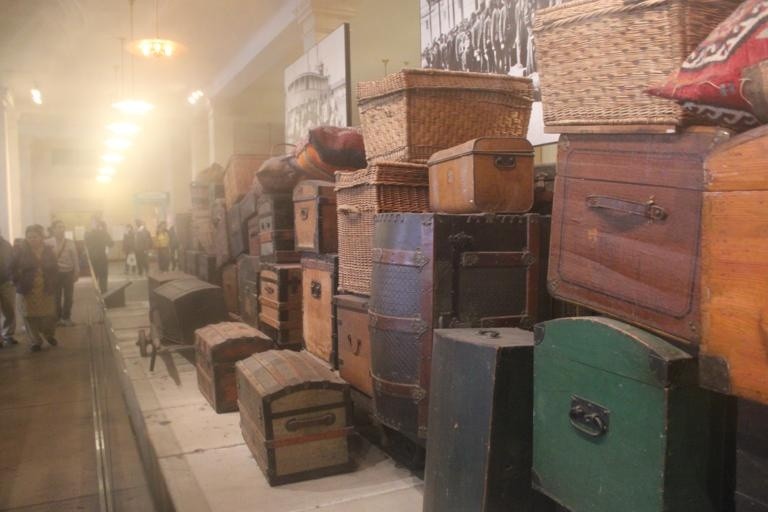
[120,218,180,280]
[47,220,81,326]
[286,89,346,156]
[0,234,19,348]
[421,0,563,81]
[11,224,61,351]
[85,215,114,294]
[11,237,32,333]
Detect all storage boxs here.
[255,261,301,346]
[548,125,733,347]
[150,271,195,322]
[152,277,228,343]
[181,164,292,324]
[194,322,274,413]
[428,138,534,214]
[235,350,353,486]
[702,124,768,407]
[298,252,338,363]
[367,209,550,447]
[530,316,734,512]
[291,178,339,254]
[421,327,534,511]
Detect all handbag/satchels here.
[252,126,366,192]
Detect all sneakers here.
[2,338,18,348]
[45,334,58,346]
[30,343,42,351]
